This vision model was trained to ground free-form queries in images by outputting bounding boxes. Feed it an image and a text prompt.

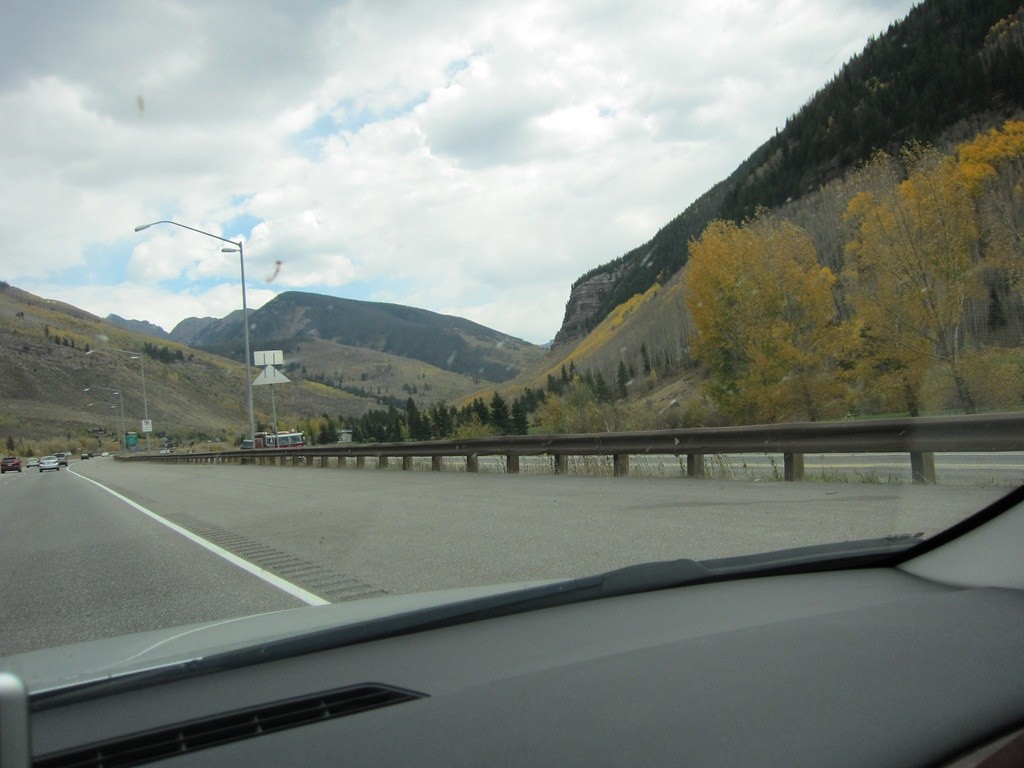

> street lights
[134,220,256,448]
[85,346,150,449]
[89,402,120,451]
[84,385,126,456]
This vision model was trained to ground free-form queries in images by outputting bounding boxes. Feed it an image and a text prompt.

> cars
[102,451,109,457]
[81,454,89,460]
[53,453,67,467]
[39,456,60,473]
[88,451,94,458]
[27,458,39,468]
[1,457,24,473]
